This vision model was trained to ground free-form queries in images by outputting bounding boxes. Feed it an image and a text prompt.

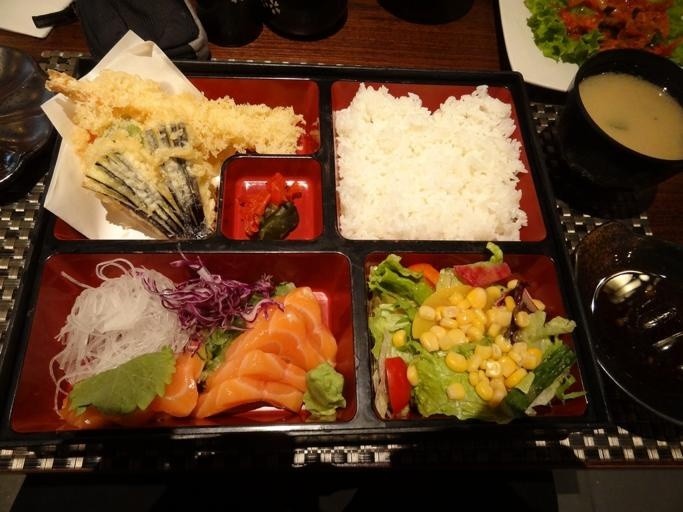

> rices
[331,81,529,240]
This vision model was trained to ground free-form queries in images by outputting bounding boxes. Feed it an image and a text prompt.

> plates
[569,219,683,437]
[0,44,53,188]
[0,56,613,442]
[498,0,683,92]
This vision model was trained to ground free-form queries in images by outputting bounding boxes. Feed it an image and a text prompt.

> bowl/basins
[558,47,683,189]
